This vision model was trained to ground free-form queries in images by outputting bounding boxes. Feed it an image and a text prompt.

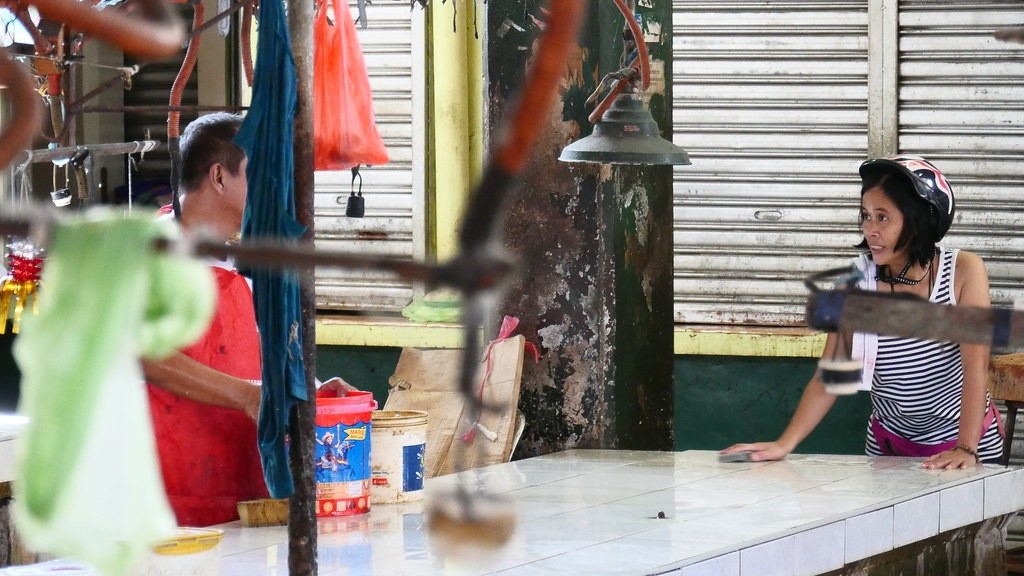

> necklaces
[874,259,932,285]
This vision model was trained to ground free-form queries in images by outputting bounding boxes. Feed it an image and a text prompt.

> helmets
[859,153,955,242]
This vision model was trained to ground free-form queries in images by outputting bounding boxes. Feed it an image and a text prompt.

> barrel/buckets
[371,409,429,505]
[314,390,378,516]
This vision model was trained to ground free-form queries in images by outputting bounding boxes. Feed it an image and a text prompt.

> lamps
[558,62,693,165]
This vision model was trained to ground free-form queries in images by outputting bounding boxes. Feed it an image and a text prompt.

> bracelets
[952,444,979,463]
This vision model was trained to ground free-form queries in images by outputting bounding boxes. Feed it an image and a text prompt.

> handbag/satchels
[873,403,1005,458]
[313,1,390,171]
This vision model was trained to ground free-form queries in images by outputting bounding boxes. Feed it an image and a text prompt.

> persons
[718,155,1006,471]
[137,111,359,527]
